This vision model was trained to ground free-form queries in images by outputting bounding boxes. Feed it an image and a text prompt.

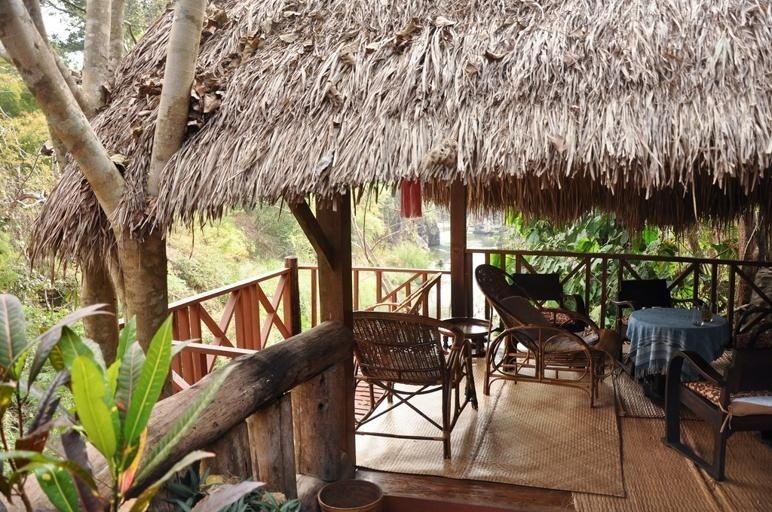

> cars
[692,302,714,326]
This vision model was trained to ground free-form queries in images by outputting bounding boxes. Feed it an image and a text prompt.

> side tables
[438,318,496,357]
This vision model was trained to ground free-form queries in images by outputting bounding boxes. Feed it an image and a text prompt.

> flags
[317,478,384,512]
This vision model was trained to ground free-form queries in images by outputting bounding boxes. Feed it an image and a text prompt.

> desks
[625,309,727,403]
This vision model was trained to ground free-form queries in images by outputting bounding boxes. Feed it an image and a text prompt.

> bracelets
[317,478,384,512]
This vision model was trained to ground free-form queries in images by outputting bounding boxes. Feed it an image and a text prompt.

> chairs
[610,279,707,381]
[660,306,771,480]
[475,265,605,408]
[352,311,478,459]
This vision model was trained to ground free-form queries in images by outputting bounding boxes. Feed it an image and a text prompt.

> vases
[317,478,384,512]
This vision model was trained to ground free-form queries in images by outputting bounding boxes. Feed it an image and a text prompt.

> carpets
[613,366,703,419]
[572,418,770,512]
[357,355,518,479]
[465,358,626,497]
